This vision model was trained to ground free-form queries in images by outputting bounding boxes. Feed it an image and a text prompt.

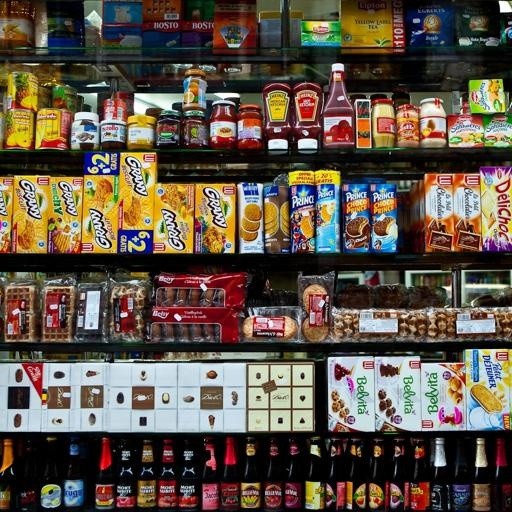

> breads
[74,281,111,346]
[40,284,77,345]
[107,280,148,343]
[150,288,224,343]
[2,280,41,345]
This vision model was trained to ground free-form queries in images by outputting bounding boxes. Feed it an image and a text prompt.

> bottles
[180,109,210,149]
[492,437,512,512]
[177,439,199,510]
[262,436,285,512]
[303,437,327,512]
[368,437,391,512]
[210,99,235,149]
[219,436,242,512]
[155,109,183,149]
[452,437,473,512]
[38,436,63,512]
[136,438,157,510]
[0,438,17,512]
[17,437,39,512]
[409,438,431,512]
[372,97,395,150]
[198,436,221,512]
[472,437,493,512]
[322,61,357,151]
[182,67,208,112]
[63,437,87,511]
[293,82,321,151]
[114,438,137,511]
[99,118,127,150]
[389,438,410,512]
[93,436,117,512]
[127,114,157,150]
[239,436,265,512]
[156,439,179,509]
[325,438,348,512]
[262,83,292,150]
[395,104,421,148]
[282,437,304,511]
[419,96,448,149]
[236,102,264,149]
[430,437,453,512]
[345,438,368,512]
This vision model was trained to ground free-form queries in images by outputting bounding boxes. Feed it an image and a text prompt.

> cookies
[124,196,142,226]
[280,200,290,238]
[332,391,356,429]
[302,284,332,343]
[242,314,298,341]
[468,383,506,417]
[300,216,314,239]
[346,216,370,247]
[202,226,224,254]
[52,232,70,253]
[448,376,462,405]
[240,204,263,241]
[93,178,113,209]
[17,219,35,249]
[374,216,395,236]
[322,204,331,223]
[263,202,280,240]
[378,389,403,430]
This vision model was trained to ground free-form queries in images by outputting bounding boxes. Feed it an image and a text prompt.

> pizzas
[160,184,182,211]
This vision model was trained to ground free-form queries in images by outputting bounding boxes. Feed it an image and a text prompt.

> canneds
[37,84,52,110]
[34,107,72,151]
[51,84,78,114]
[70,111,100,151]
[5,69,39,113]
[2,105,36,150]
[77,95,84,111]
